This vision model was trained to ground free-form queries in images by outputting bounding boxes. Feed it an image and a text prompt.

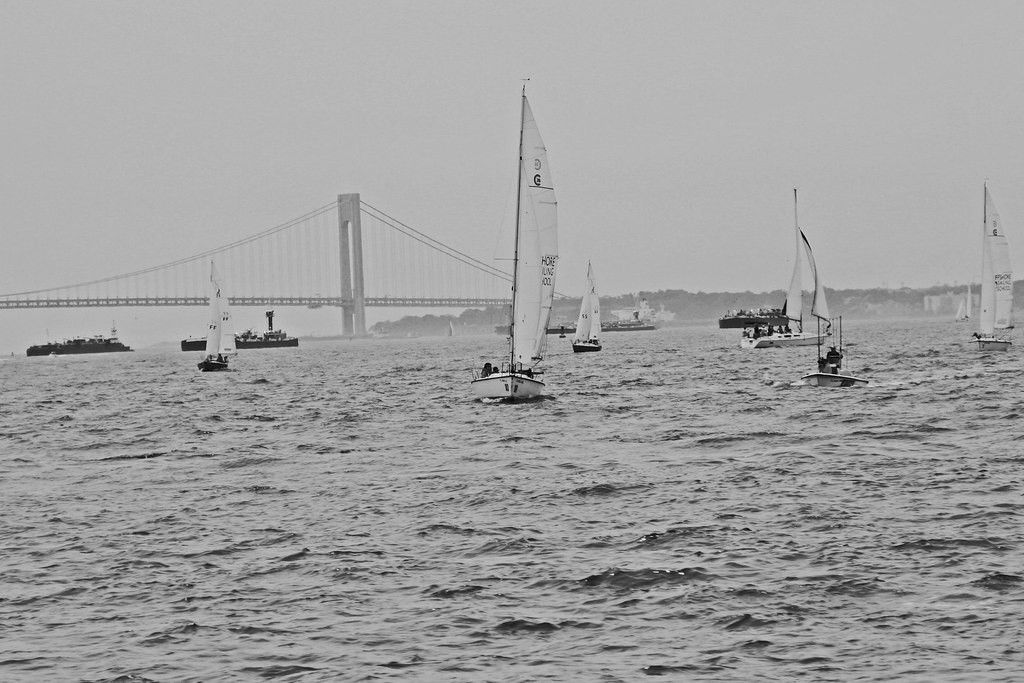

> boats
[180,310,300,354]
[491,318,656,336]
[26,333,135,356]
[718,307,790,329]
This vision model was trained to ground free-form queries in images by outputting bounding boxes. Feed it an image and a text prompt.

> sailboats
[799,226,869,390]
[740,187,834,353]
[572,259,605,352]
[966,180,1014,352]
[469,77,561,405]
[197,257,233,372]
[956,286,973,322]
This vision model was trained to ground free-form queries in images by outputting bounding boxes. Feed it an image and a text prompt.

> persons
[217,354,229,363]
[481,363,499,378]
[743,323,792,339]
[827,346,843,360]
